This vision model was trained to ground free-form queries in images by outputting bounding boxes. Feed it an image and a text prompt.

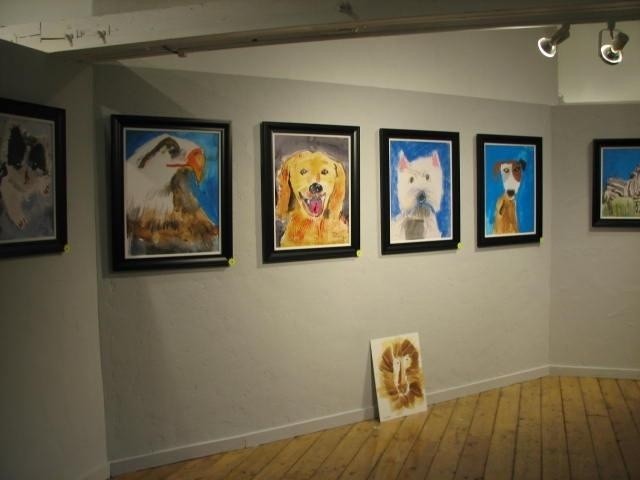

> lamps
[537,22,575,57]
[599,18,630,65]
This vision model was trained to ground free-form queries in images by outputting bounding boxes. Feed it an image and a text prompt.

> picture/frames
[259,121,361,264]
[0,97,67,259]
[593,139,640,227]
[379,128,461,254]
[109,114,233,271]
[476,132,543,248]
